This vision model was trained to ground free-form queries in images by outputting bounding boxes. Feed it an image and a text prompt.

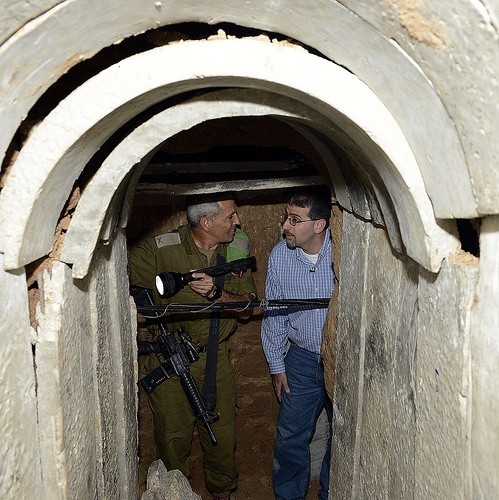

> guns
[129,284,220,447]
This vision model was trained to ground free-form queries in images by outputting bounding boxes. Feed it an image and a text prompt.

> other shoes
[212,490,231,500]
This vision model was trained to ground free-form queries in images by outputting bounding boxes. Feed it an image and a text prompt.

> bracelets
[205,285,216,298]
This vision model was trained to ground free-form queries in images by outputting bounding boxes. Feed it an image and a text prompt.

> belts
[195,346,207,353]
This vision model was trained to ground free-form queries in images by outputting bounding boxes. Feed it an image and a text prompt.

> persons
[128,195,257,500]
[261,187,337,500]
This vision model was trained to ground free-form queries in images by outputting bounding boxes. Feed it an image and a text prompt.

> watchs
[207,284,223,302]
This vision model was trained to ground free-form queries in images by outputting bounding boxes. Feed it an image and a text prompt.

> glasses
[279,214,320,226]
